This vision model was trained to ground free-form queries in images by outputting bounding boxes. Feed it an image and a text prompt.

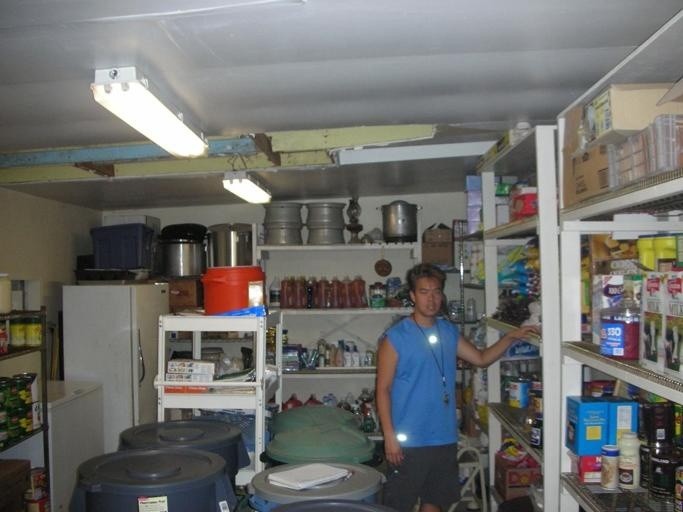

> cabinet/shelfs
[553,11,683,512]
[155,312,284,484]
[255,238,421,443]
[1,305,58,510]
[482,123,557,511]
[446,219,487,480]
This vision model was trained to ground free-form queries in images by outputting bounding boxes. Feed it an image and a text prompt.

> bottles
[466,296,476,320]
[618,430,642,489]
[269,273,367,309]
[0,272,13,316]
[0,311,43,359]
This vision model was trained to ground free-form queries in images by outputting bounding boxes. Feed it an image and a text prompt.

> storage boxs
[421,223,456,267]
[494,440,542,507]
[565,395,638,484]
[561,74,683,208]
[89,222,158,277]
[589,258,682,384]
[166,277,202,312]
[0,459,33,508]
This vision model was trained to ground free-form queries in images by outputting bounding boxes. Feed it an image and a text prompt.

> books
[266,461,354,492]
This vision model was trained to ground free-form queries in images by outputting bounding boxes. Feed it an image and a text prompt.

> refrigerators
[61,282,169,455]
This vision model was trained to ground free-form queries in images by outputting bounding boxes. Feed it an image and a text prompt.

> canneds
[25,316,43,348]
[9,316,25,352]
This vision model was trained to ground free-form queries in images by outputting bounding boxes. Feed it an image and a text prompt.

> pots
[201,224,252,266]
[375,199,422,242]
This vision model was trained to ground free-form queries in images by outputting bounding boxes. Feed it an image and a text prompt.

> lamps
[222,151,275,206]
[91,67,207,160]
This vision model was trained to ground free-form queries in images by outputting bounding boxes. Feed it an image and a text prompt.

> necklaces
[410,313,450,410]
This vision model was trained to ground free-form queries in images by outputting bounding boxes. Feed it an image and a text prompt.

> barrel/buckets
[206,222,252,267]
[117,418,251,468]
[250,463,387,511]
[68,448,239,511]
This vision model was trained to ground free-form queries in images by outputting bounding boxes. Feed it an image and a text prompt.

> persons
[374,261,540,512]
